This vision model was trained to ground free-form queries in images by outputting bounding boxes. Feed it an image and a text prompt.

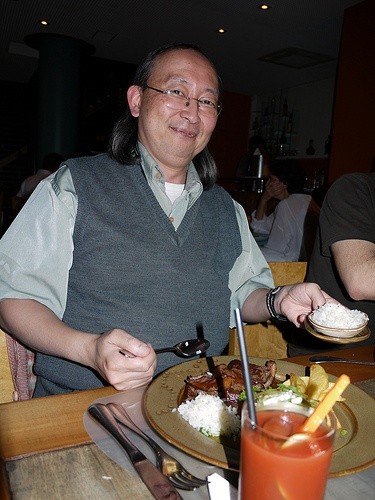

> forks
[107,402,211,491]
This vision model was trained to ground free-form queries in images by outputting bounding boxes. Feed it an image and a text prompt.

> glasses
[141,84,224,118]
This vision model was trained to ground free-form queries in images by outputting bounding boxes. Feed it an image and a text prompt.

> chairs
[0,329,38,405]
[227,261,309,360]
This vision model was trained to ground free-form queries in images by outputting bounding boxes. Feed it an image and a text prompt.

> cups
[238,391,336,500]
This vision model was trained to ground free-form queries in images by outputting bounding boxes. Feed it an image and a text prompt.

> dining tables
[0,345,375,500]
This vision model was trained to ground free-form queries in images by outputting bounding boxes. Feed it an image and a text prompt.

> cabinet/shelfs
[248,94,298,156]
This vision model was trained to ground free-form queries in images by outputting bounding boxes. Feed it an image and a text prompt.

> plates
[305,319,371,343]
[143,355,375,478]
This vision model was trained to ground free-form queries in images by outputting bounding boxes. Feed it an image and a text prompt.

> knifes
[87,403,184,500]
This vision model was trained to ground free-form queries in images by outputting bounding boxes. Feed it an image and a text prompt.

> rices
[312,302,369,329]
[170,391,241,436]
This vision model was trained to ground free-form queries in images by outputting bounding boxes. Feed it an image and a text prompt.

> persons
[288,171,375,356]
[17,153,64,197]
[0,42,352,399]
[251,158,320,262]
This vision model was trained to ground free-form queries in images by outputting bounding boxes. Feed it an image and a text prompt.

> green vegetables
[238,382,321,409]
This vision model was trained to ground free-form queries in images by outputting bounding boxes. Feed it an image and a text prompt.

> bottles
[305,138,316,155]
[324,134,330,155]
[242,162,327,193]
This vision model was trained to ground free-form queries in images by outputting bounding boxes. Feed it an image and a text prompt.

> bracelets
[266,285,287,321]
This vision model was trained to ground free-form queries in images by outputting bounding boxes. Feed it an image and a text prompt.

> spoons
[118,338,210,359]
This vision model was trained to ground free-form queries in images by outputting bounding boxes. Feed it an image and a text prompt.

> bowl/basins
[308,310,369,338]
